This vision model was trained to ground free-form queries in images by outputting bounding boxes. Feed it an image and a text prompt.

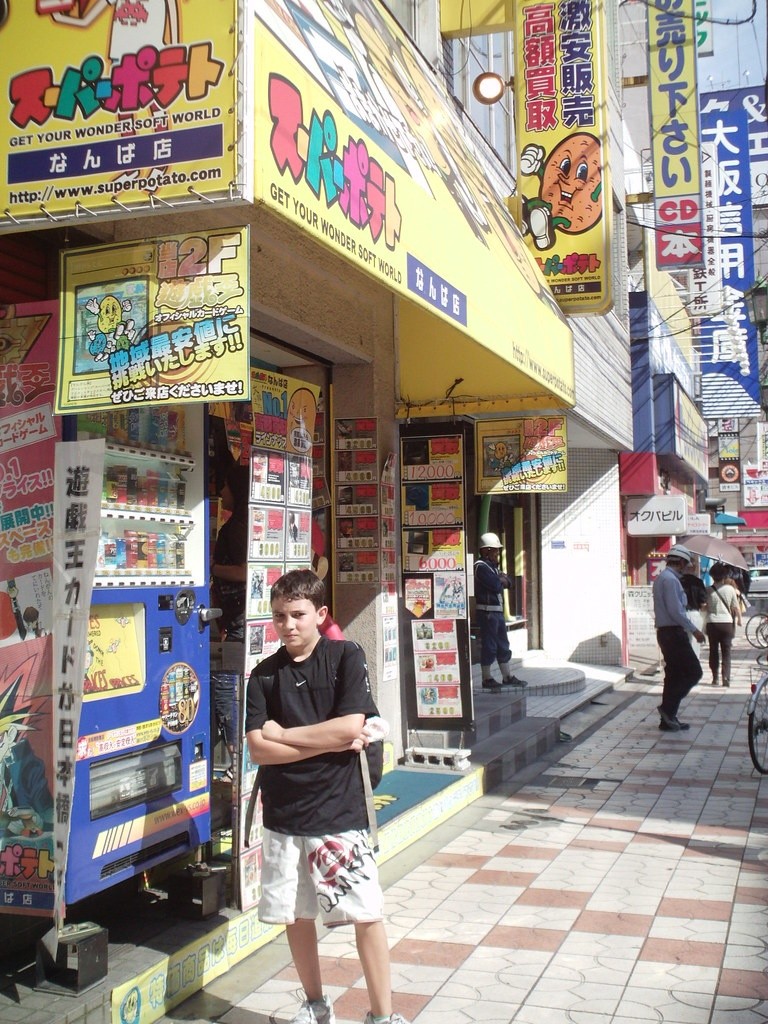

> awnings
[714,513,747,526]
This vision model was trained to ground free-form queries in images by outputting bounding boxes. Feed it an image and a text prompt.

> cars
[747,567,768,593]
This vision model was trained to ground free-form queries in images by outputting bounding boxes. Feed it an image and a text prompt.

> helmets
[478,533,503,549]
[667,544,694,568]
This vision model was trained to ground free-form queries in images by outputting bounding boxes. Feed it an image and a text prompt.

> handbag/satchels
[739,600,746,614]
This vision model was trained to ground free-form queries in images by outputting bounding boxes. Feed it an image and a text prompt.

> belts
[684,608,699,612]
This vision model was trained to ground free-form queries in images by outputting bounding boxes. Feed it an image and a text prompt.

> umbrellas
[676,535,749,571]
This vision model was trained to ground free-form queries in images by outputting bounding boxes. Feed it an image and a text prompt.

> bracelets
[211,563,216,576]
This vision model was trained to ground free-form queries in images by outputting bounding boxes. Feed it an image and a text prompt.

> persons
[245,569,412,1024]
[210,464,250,784]
[653,544,751,731]
[474,533,528,688]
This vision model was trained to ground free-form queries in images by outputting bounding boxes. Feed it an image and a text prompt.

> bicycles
[744,613,768,776]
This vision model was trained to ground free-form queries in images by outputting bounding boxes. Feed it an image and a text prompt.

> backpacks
[253,639,386,791]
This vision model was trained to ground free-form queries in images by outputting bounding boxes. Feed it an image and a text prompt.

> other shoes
[481,679,502,689]
[712,680,719,684]
[658,718,690,730]
[502,676,528,687]
[723,675,729,686]
[657,705,681,732]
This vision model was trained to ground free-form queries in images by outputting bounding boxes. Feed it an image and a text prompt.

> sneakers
[364,1010,411,1024]
[268,989,336,1024]
